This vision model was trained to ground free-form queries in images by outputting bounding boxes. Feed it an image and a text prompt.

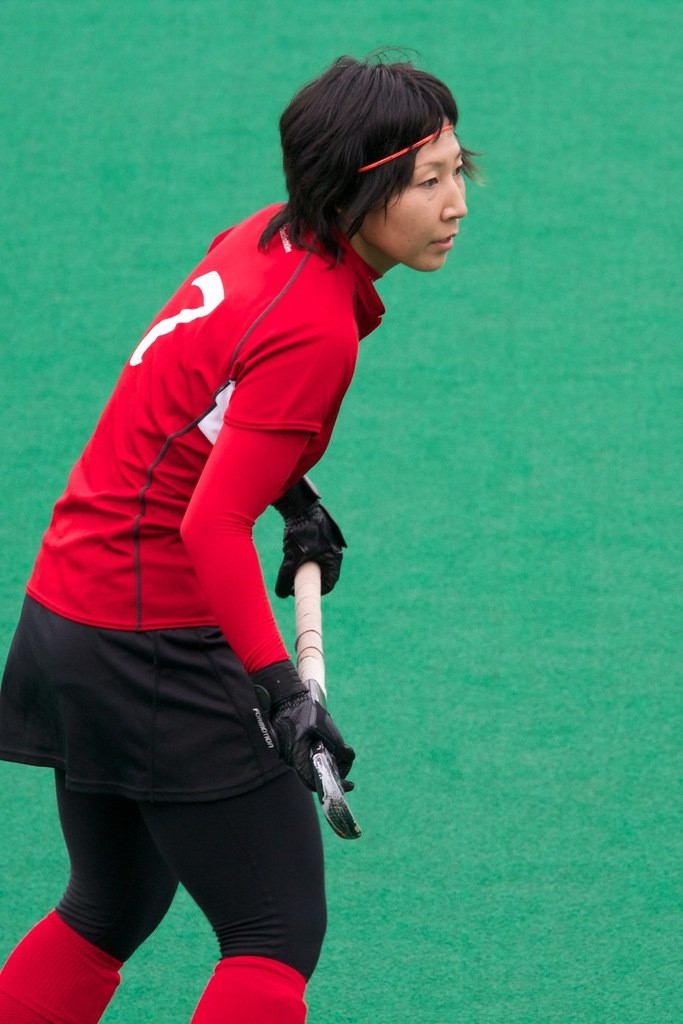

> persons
[0,52,472,1024]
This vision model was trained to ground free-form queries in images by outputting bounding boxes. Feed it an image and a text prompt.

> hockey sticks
[292,560,365,841]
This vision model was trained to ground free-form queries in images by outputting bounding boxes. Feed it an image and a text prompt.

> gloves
[247,659,356,793]
[269,475,348,599]
